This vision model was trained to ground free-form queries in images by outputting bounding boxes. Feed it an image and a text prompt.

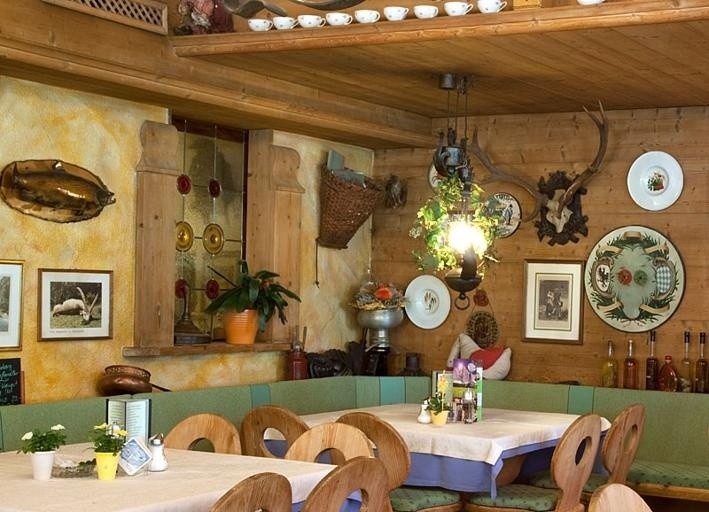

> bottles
[694,332,708,392]
[463,391,474,424]
[657,354,680,392]
[623,339,639,388]
[147,437,168,471]
[601,340,619,387]
[680,330,693,391]
[418,399,431,423]
[645,329,658,389]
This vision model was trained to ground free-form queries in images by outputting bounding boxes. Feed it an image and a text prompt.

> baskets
[318,165,385,250]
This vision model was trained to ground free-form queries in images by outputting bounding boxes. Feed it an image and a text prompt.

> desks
[1,441,339,512]
[295,404,613,511]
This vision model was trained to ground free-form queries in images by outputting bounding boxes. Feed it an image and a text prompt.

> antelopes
[52,284,101,324]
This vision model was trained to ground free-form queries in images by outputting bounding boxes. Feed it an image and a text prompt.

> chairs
[533,403,652,512]
[464,413,602,512]
[284,423,374,480]
[328,412,462,512]
[239,406,318,462]
[161,414,244,457]
[208,472,292,512]
[304,457,390,509]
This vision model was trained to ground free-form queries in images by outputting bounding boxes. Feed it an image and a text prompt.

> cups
[288,351,308,380]
[405,352,420,370]
[245,0,510,34]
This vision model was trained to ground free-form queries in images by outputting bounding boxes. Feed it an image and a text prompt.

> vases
[355,307,404,353]
[31,451,55,481]
[95,450,119,480]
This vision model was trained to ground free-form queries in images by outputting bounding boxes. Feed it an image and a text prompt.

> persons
[505,204,513,224]
[554,288,563,319]
[546,290,554,317]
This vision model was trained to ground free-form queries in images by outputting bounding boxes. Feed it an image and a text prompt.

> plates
[428,148,472,196]
[403,275,450,329]
[627,150,683,210]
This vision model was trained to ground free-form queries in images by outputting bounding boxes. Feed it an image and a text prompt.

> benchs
[591,386,709,512]
[1,375,356,452]
[356,375,592,413]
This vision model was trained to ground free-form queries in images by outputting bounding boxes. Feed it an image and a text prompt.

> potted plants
[204,259,303,345]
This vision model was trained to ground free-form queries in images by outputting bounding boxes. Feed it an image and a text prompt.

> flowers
[15,423,70,454]
[347,275,411,311]
[87,422,129,457]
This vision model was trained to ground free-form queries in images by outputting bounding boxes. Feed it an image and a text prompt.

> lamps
[434,73,484,312]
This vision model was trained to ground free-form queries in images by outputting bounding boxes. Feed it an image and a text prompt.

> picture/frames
[37,268,113,341]
[522,258,586,345]
[0,258,25,352]
[583,225,686,333]
